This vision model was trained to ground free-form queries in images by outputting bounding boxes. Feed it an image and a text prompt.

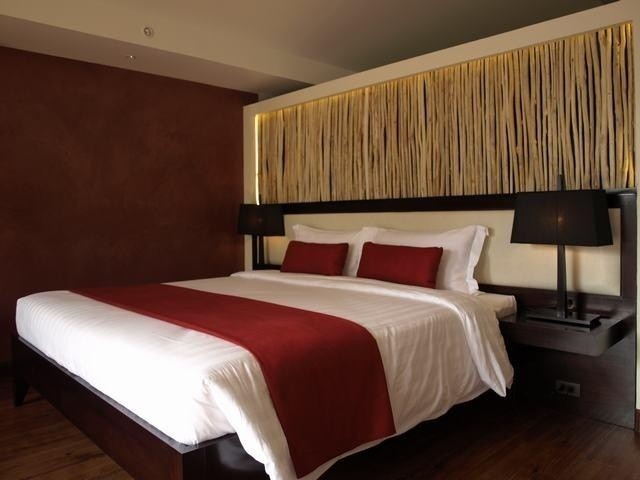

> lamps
[238,201,285,266]
[508,190,615,330]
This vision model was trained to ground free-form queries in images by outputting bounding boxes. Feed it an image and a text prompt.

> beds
[8,271,519,480]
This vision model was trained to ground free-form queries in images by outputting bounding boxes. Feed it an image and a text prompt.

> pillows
[279,222,491,297]
